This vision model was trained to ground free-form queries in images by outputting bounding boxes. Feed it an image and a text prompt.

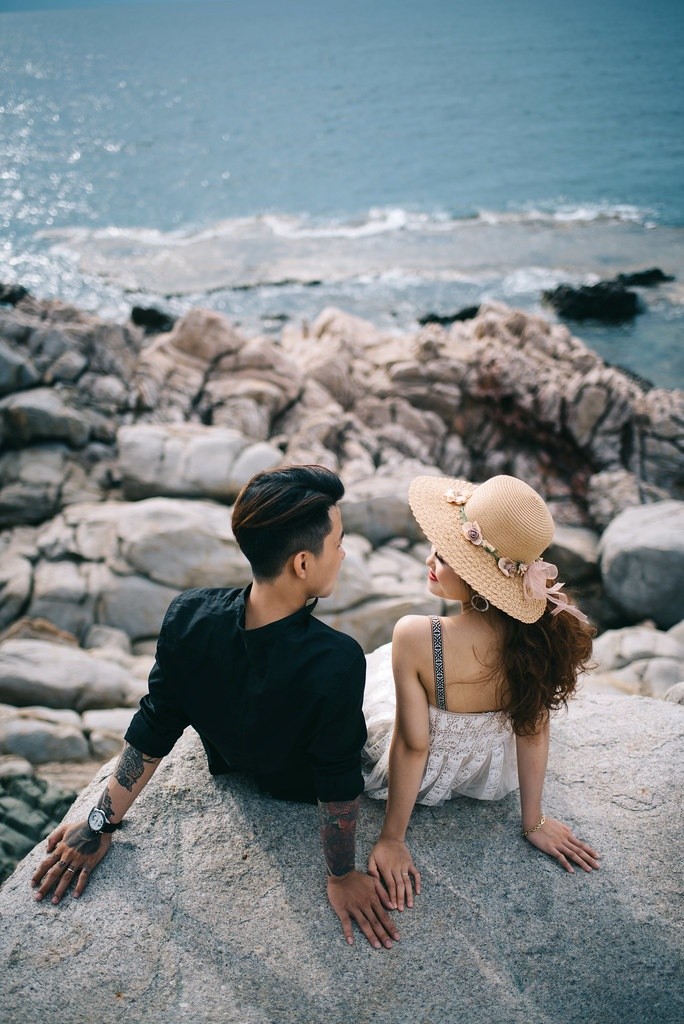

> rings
[401,872,408,876]
[67,867,76,874]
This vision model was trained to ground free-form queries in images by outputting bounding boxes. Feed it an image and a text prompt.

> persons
[28,465,401,950]
[364,473,603,914]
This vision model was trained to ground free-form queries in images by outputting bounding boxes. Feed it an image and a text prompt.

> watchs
[88,806,123,833]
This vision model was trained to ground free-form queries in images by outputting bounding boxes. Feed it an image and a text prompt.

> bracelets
[521,814,545,836]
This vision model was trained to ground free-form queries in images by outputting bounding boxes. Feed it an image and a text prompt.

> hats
[408,474,589,624]
